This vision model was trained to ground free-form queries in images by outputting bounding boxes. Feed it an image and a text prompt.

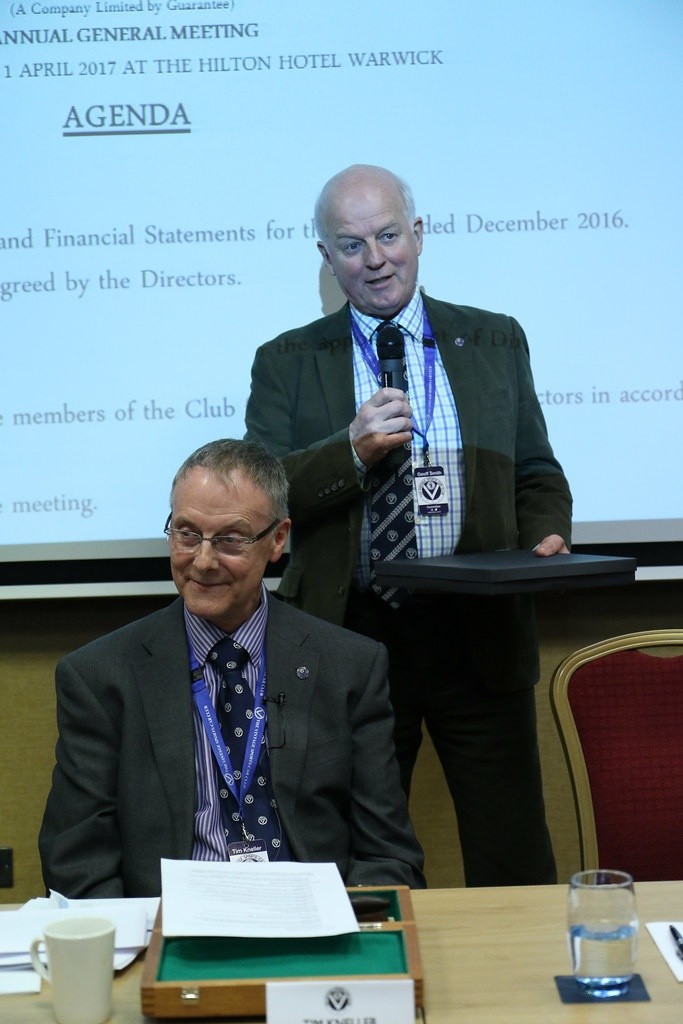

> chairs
[548,626,683,886]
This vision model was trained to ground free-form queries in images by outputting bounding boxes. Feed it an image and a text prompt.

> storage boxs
[139,885,426,1018]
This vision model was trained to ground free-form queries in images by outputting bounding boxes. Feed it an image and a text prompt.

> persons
[243,165,574,890]
[38,437,428,901]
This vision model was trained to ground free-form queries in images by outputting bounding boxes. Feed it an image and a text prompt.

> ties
[204,636,257,848]
[364,320,420,604]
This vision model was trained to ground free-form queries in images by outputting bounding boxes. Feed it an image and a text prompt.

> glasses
[163,511,281,556]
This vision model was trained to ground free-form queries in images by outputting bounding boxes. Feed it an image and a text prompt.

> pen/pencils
[668,923,683,962]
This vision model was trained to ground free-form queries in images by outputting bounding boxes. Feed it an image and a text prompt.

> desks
[0,879,683,1024]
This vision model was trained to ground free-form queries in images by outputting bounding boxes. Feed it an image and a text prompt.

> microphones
[376,326,406,465]
[278,692,287,710]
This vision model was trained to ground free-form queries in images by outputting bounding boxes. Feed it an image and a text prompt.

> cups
[569,870,637,997]
[31,917,115,1024]
